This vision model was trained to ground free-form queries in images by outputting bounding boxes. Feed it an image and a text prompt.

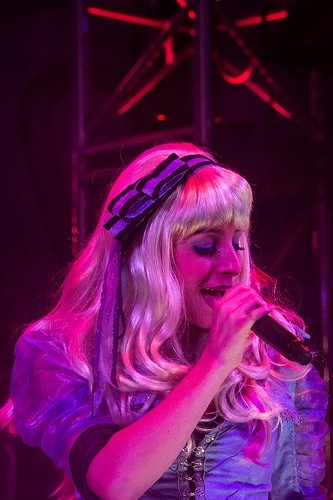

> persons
[1,141,331,500]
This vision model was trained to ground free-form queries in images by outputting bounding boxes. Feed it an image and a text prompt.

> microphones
[250,313,313,365]
